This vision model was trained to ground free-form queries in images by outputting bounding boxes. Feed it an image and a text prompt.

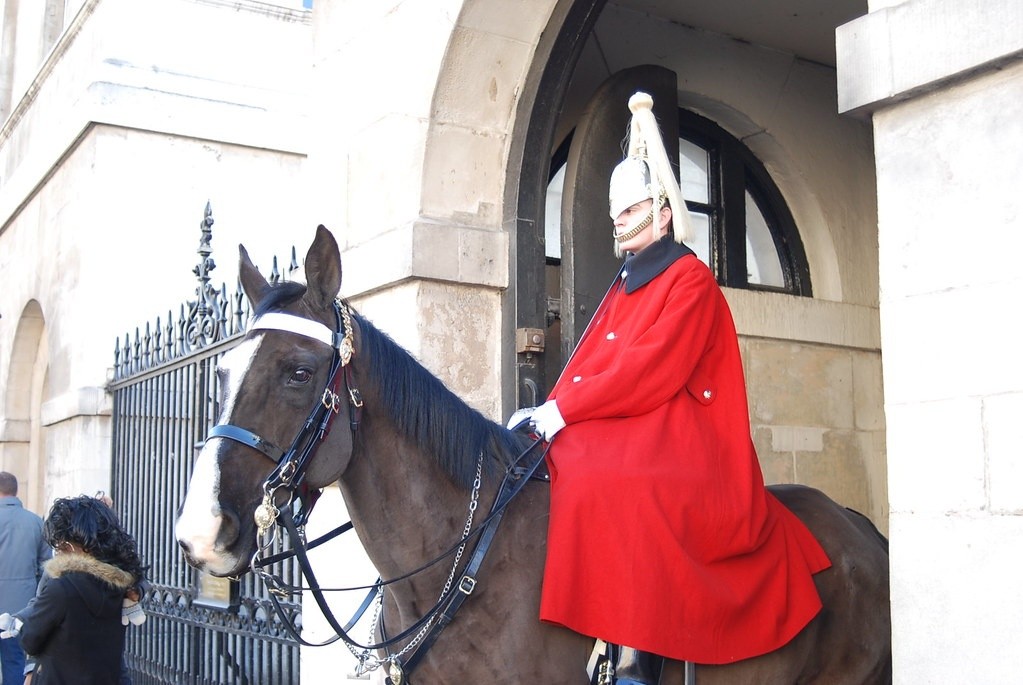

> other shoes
[616,641,665,685]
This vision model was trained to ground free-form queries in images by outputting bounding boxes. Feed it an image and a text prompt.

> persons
[0,469,53,685]
[12,494,151,685]
[526,153,754,685]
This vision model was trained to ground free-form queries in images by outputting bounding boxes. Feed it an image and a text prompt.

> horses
[169,224,891,683]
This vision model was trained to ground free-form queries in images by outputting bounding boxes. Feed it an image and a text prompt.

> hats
[607,90,697,244]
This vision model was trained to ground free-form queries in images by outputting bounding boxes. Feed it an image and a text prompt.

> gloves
[0,613,23,638]
[507,405,537,431]
[531,400,566,442]
[122,599,146,626]
